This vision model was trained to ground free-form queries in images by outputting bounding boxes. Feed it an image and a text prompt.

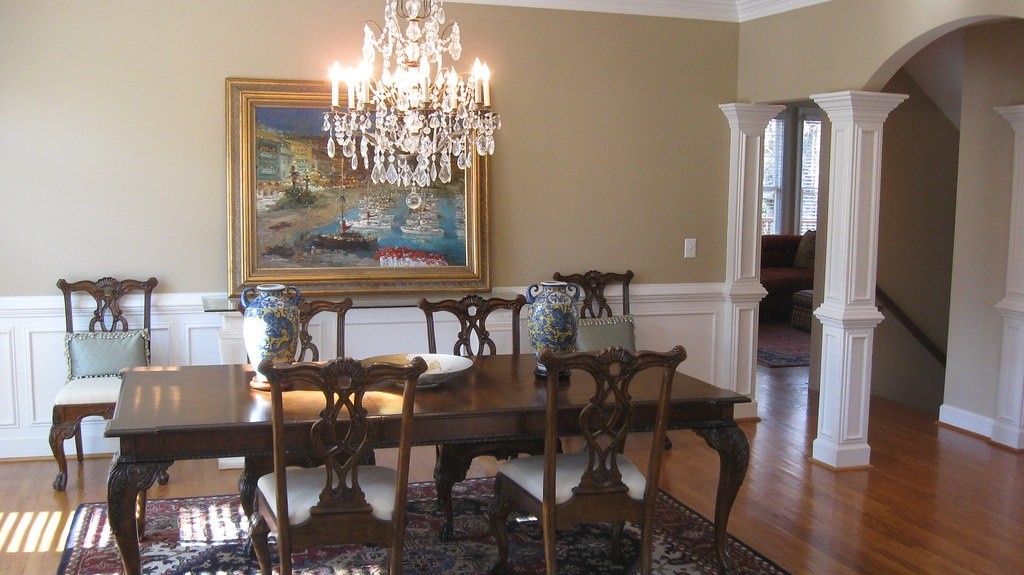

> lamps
[319,0,501,210]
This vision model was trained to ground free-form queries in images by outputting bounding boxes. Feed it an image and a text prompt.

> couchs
[760,230,814,318]
[791,289,812,333]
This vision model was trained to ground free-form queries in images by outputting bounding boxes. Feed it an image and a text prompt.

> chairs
[47,277,168,496]
[480,345,692,573]
[548,269,678,452]
[414,292,562,540]
[227,294,376,535]
[252,355,431,575]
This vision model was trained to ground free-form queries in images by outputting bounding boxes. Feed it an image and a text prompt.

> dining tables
[97,341,757,572]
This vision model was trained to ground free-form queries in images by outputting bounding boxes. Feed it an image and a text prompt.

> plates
[363,352,475,388]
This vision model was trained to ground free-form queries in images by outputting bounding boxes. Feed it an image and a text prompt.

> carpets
[55,480,792,575]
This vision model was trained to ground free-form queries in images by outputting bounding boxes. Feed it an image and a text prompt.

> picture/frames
[225,74,494,300]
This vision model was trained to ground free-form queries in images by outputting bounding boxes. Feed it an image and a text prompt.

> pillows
[793,229,816,270]
